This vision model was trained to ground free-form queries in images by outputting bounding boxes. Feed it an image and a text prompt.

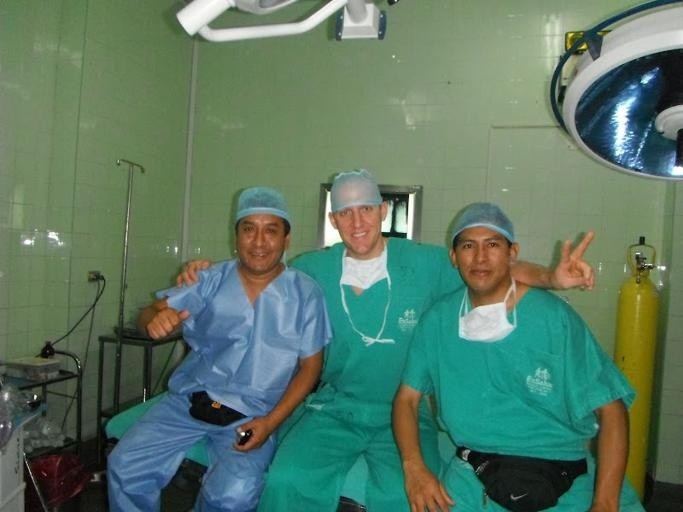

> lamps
[548,1,683,184]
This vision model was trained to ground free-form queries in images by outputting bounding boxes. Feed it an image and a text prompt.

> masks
[457,300,516,342]
[339,251,390,289]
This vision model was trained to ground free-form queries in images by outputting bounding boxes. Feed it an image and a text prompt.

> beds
[104,388,457,512]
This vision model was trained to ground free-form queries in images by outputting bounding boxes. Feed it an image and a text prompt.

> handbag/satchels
[187,389,247,427]
[454,445,589,512]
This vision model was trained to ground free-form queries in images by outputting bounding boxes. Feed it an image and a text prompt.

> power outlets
[88,270,101,284]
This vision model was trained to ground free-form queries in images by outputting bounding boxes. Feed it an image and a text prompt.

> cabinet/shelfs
[0,348,86,463]
[95,331,188,465]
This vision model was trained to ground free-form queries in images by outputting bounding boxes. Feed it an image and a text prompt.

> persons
[384,201,646,512]
[165,164,598,511]
[90,184,336,512]
